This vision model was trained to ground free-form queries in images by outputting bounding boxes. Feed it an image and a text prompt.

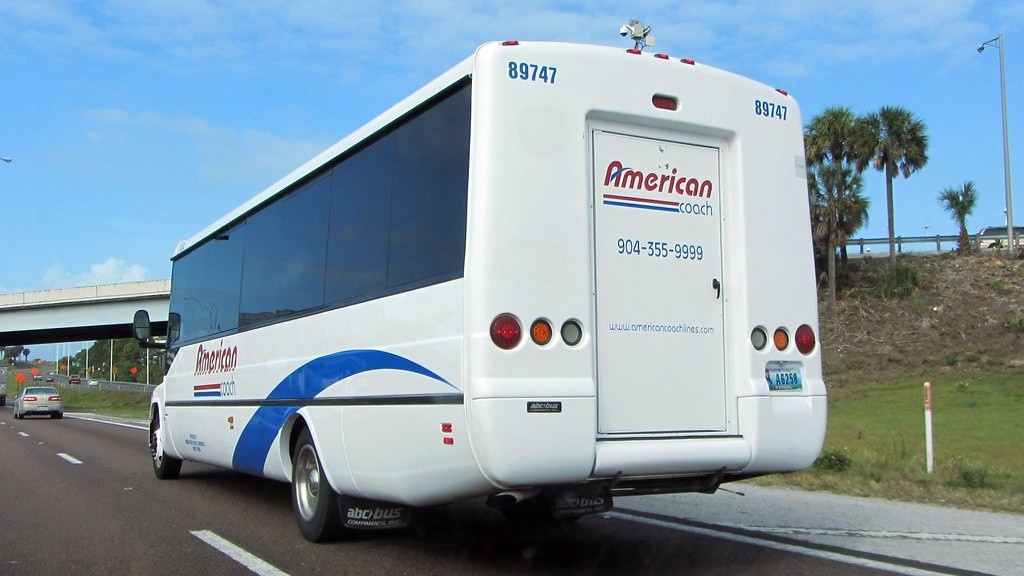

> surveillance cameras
[620,27,628,37]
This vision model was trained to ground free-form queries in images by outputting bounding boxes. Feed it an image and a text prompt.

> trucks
[0,366,9,406]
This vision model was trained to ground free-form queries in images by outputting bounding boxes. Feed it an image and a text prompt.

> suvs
[68,374,81,384]
[968,226,1024,250]
[33,374,43,381]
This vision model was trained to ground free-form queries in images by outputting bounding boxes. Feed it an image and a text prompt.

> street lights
[977,32,1015,257]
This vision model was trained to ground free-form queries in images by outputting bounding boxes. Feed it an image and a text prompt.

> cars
[13,386,64,420]
[45,377,54,382]
[87,379,99,387]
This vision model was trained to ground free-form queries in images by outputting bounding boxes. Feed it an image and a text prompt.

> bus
[130,20,830,546]
